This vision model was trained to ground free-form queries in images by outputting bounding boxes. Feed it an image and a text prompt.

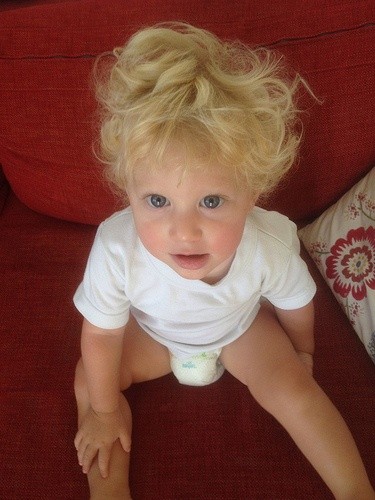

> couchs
[0,0,375,500]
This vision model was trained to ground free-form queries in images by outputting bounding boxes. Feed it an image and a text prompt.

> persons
[73,20,375,500]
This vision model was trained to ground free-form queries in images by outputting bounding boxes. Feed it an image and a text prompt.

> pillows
[296,168,375,363]
